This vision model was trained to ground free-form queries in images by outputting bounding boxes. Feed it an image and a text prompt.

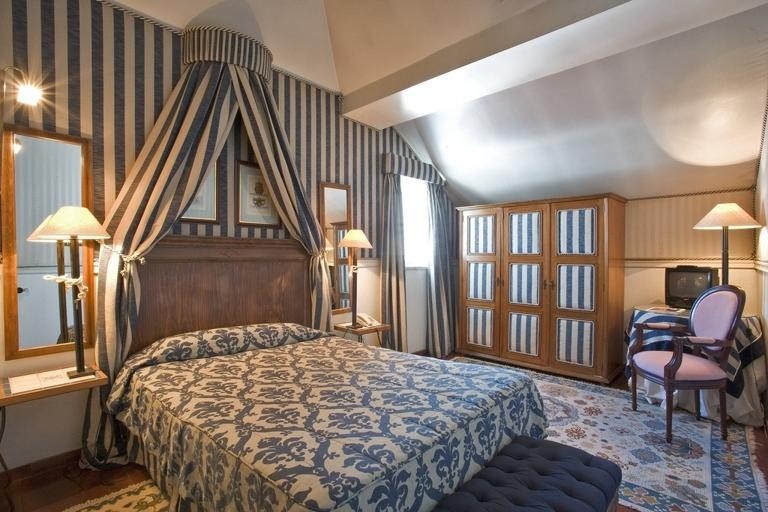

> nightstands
[336,318,391,346]
[2,363,113,511]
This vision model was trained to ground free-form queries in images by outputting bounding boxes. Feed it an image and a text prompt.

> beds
[124,232,546,510]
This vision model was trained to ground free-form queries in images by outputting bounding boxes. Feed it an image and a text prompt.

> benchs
[430,433,621,510]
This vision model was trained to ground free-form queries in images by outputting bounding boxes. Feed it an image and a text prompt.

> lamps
[691,202,762,283]
[1,64,44,116]
[338,229,373,328]
[38,206,108,379]
[24,219,72,344]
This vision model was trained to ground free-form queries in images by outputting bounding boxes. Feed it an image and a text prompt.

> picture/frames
[180,154,221,224]
[236,158,283,230]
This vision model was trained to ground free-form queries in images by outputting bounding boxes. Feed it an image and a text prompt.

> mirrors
[317,182,355,314]
[1,123,97,361]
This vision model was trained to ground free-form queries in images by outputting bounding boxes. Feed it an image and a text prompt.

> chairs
[628,283,746,443]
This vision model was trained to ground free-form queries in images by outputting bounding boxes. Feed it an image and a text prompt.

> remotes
[677,265,697,268]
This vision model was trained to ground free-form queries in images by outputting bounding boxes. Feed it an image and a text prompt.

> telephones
[356,313,381,328]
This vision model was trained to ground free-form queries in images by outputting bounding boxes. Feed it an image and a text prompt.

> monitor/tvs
[665,267,719,310]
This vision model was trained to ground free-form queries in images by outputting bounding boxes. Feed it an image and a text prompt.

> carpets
[63,482,187,512]
[446,353,768,512]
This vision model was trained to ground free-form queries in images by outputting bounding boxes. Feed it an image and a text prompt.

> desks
[625,301,767,427]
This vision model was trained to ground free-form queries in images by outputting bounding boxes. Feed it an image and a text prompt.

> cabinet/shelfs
[331,222,350,308]
[452,192,627,386]
